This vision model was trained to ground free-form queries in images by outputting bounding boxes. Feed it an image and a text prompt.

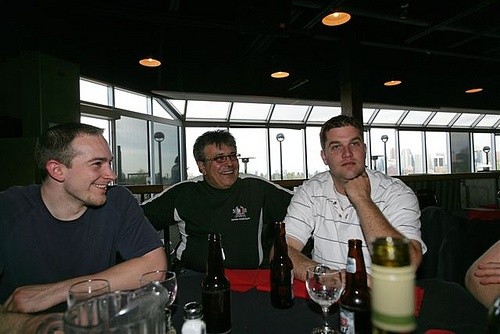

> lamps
[383,72,402,86]
[464,84,483,94]
[320,8,352,27]
[270,65,290,79]
[138,48,161,68]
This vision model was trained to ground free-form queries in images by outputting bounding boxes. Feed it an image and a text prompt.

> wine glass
[304,263,343,334]
[138,270,178,334]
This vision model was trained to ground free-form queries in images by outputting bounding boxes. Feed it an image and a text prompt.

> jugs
[62,289,167,334]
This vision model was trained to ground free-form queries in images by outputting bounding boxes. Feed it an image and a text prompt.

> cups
[66,279,110,310]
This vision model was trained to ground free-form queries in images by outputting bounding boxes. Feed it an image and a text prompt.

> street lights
[483,146,490,165]
[381,135,388,175]
[239,156,254,174]
[371,156,384,171]
[155,132,164,184]
[276,133,284,180]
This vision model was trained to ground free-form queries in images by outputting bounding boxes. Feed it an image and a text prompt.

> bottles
[180,301,206,334]
[368,237,420,334]
[338,239,373,334]
[201,234,232,334]
[271,223,295,309]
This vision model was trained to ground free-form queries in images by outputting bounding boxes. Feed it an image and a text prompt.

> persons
[0,123,169,334]
[269,116,427,293]
[140,129,314,273]
[465,239,500,310]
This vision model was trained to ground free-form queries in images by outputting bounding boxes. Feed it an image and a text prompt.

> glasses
[205,154,241,163]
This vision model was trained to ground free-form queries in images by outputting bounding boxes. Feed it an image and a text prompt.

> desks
[173,272,341,334]
[448,204,500,224]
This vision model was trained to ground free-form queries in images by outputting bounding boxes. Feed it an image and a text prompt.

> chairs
[414,189,439,207]
